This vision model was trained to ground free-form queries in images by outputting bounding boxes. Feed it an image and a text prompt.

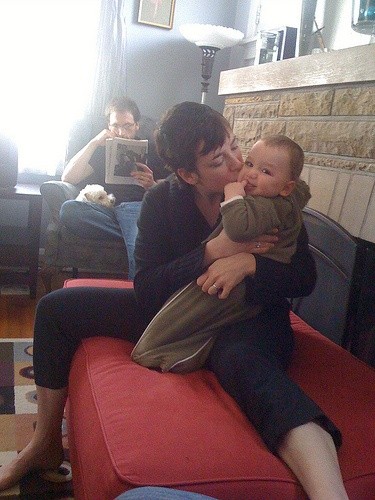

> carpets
[0,337,74,500]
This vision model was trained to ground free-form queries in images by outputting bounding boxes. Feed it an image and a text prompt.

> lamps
[179,24,244,104]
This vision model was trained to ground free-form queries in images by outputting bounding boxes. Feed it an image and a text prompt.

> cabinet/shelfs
[240,26,297,66]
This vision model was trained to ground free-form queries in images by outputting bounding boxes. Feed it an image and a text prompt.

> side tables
[0,183,42,299]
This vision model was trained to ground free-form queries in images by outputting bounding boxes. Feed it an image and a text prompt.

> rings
[212,284,220,289]
[255,241,262,248]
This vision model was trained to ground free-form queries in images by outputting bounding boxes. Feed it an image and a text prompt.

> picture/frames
[137,0,176,30]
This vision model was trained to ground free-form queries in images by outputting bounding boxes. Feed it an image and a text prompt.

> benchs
[61,276,374,499]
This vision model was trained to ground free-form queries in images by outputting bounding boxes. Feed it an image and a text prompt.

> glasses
[110,122,135,131]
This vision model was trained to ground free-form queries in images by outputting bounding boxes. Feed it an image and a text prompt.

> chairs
[40,116,171,294]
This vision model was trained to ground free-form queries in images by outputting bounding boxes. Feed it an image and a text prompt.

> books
[104,138,148,185]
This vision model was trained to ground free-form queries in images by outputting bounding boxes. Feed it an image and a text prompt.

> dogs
[73,182,117,210]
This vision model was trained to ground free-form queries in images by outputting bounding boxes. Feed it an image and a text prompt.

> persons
[59,98,167,281]
[131,135,312,373]
[0,101,349,500]
